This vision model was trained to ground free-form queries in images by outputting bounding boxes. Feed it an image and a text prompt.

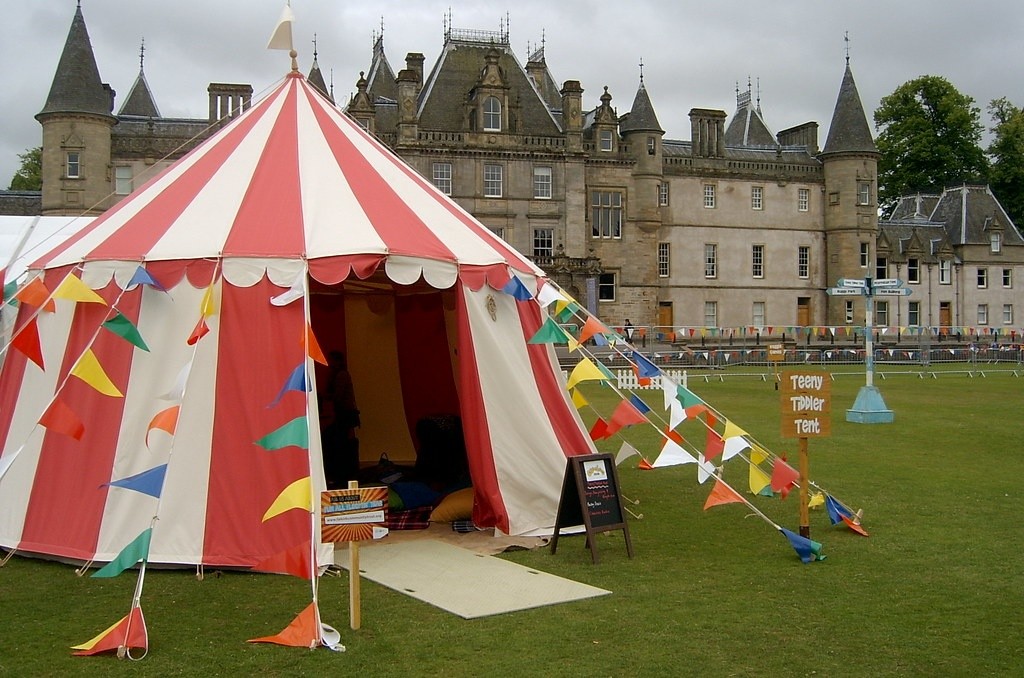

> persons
[624,319,634,343]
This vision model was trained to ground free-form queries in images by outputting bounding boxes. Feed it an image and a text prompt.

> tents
[0,48,601,583]
[1,215,98,272]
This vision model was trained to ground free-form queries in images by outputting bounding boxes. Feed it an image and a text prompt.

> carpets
[335,539,612,620]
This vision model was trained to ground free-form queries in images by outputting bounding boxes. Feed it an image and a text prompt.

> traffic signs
[826,277,913,296]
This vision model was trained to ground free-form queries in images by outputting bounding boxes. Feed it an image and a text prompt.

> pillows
[391,482,444,510]
[427,487,474,523]
[358,482,403,509]
